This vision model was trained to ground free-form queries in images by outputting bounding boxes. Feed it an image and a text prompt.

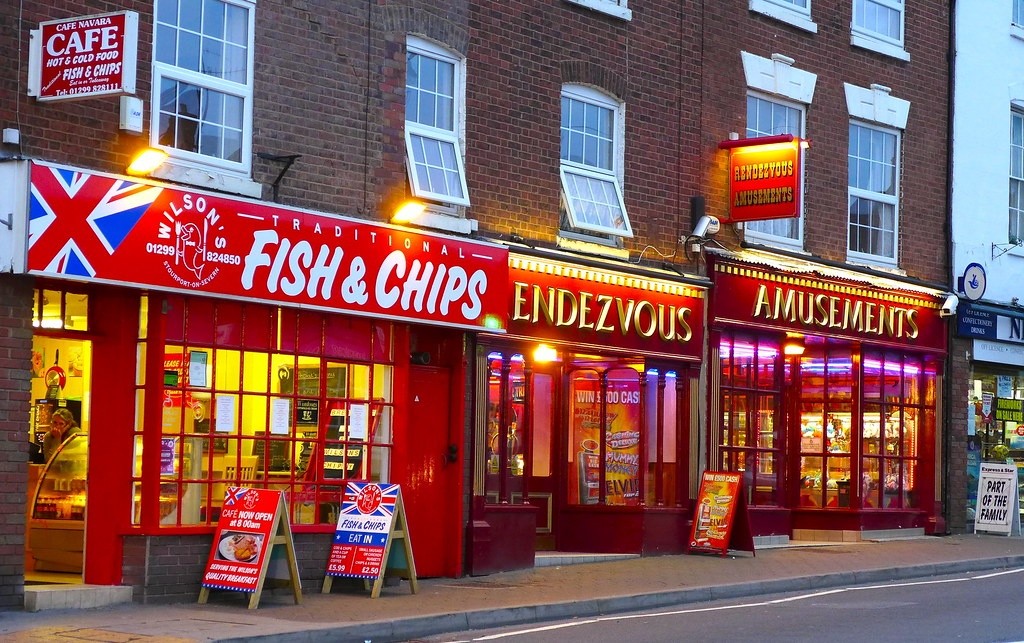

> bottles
[56,497,71,520]
[71,499,86,520]
[43,498,49,519]
[37,497,42,519]
[49,498,56,520]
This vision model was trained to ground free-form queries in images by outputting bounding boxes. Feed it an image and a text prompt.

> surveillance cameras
[692,216,711,238]
[942,296,959,316]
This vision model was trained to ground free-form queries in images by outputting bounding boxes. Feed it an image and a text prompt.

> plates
[219,534,260,561]
[583,439,599,451]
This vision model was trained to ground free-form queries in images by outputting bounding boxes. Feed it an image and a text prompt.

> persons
[43,408,82,474]
